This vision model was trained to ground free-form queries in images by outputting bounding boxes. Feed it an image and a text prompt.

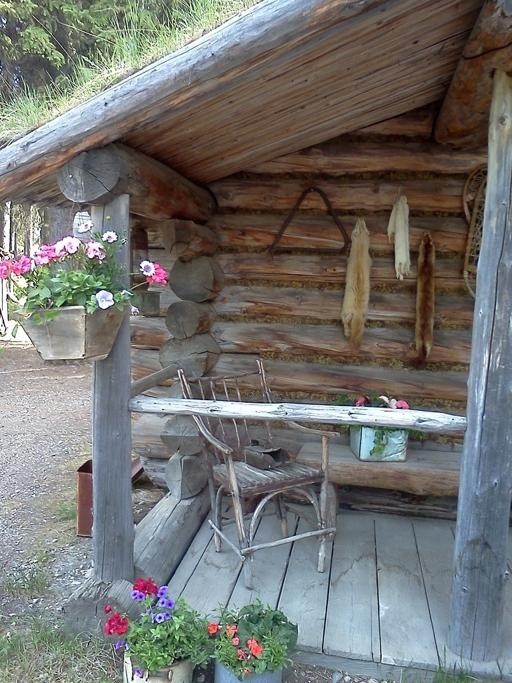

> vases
[11,304,125,364]
[349,428,411,462]
[75,458,145,538]
[123,643,283,683]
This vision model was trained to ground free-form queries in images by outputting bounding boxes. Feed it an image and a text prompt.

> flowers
[102,576,302,681]
[348,394,424,456]
[0,213,169,336]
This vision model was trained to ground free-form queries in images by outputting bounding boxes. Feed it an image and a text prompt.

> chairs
[176,357,342,589]
